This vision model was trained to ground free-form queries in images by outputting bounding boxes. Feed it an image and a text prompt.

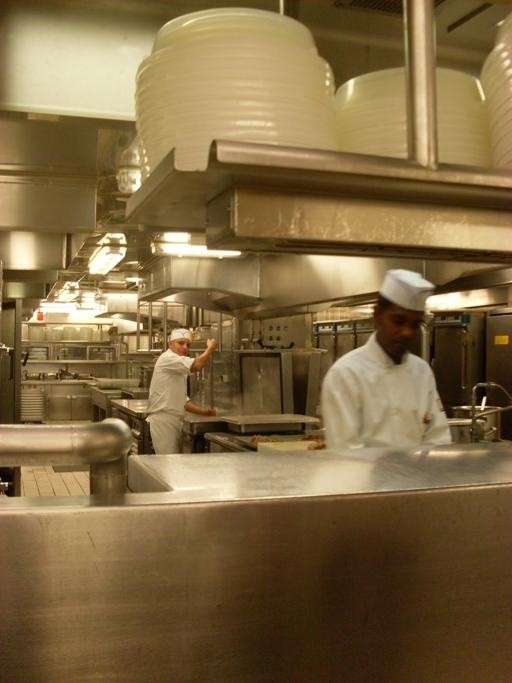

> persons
[319,269,453,449]
[136,327,219,456]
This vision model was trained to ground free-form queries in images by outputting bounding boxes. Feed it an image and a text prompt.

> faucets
[469,381,512,443]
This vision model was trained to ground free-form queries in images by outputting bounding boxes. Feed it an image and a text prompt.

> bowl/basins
[132,8,512,182]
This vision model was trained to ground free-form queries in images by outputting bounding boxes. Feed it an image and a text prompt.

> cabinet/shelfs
[49,393,94,422]
[18,318,120,362]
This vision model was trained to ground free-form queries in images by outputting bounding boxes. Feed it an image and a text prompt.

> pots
[449,406,501,442]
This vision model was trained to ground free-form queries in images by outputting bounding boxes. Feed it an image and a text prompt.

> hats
[378,267,438,314]
[169,327,193,342]
